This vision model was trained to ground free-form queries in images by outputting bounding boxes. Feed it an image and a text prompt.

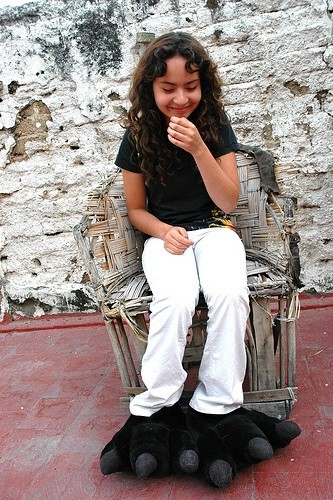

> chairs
[74,143,306,419]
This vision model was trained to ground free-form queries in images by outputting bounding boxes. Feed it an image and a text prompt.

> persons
[97,32,301,487]
